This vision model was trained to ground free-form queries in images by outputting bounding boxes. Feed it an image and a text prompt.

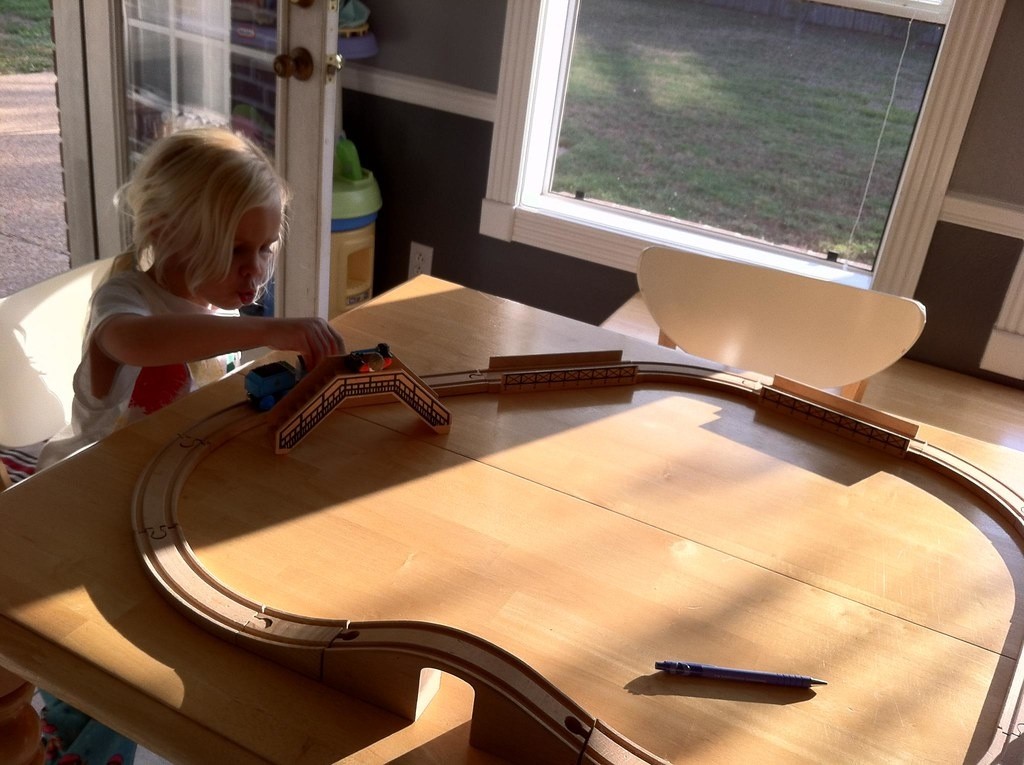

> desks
[1,272,1024,765]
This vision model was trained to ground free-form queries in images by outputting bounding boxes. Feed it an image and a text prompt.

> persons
[35,128,345,765]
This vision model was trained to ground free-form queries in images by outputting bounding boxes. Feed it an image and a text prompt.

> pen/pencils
[653,661,829,689]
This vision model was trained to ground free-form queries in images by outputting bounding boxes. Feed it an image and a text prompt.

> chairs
[635,244,925,399]
